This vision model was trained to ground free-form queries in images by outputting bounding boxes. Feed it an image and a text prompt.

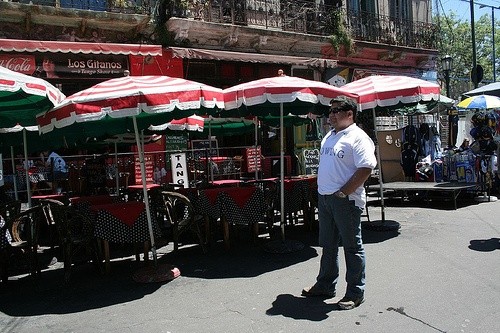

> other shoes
[337,295,366,309]
[302,285,337,298]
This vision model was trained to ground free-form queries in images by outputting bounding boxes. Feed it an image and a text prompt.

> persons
[42,54,59,78]
[302,95,377,310]
[40,148,69,194]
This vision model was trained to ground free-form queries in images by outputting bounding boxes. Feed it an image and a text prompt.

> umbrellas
[36,70,226,260]
[203,69,362,235]
[457,95,500,111]
[340,75,500,139]
[0,65,66,209]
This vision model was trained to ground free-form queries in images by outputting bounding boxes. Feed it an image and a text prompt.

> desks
[264,174,317,230]
[184,185,264,242]
[69,195,163,274]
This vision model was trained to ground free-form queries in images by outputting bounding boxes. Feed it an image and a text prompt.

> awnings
[169,47,337,69]
[0,38,162,56]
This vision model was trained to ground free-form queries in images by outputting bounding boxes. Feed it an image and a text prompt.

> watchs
[339,191,346,198]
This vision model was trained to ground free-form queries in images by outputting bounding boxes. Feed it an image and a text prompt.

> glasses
[328,107,348,114]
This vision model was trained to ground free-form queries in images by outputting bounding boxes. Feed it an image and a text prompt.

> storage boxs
[374,129,408,181]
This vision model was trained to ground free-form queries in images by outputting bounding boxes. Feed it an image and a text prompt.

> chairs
[0,179,371,279]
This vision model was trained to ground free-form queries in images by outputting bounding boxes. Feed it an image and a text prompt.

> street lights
[440,54,454,150]
